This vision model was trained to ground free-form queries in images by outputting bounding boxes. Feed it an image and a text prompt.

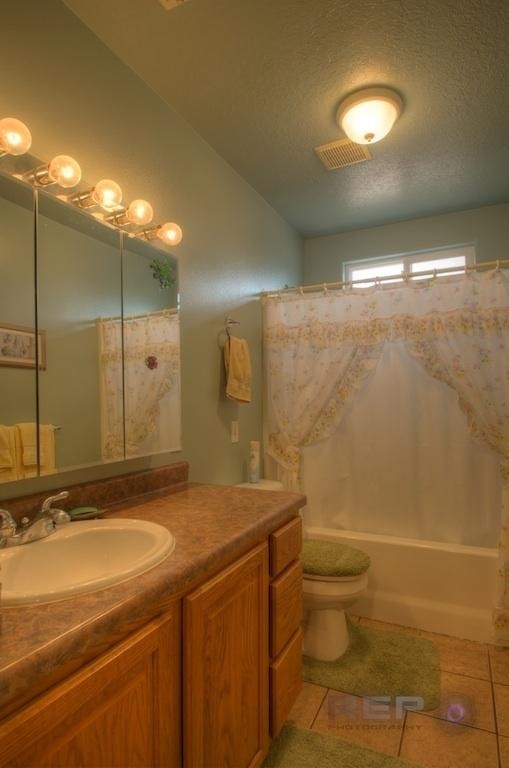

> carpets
[301,616,443,714]
[267,719,428,768]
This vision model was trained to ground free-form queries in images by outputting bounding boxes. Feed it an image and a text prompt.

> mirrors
[0,171,183,502]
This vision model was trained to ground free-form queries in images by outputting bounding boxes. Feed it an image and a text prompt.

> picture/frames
[2,319,48,374]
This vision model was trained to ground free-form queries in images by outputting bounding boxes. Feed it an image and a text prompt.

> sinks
[0,519,175,608]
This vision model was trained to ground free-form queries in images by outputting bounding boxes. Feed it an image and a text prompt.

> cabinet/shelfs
[0,604,179,767]
[180,537,275,768]
[267,511,308,735]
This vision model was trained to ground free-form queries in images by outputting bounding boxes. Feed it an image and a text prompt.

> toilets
[233,480,370,662]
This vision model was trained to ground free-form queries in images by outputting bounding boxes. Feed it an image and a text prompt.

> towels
[225,335,256,409]
[16,420,56,483]
[1,423,21,485]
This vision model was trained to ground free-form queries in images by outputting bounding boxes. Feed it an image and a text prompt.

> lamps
[327,82,412,148]
[0,113,186,248]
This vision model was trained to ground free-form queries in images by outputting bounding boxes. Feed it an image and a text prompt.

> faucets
[22,508,69,545]
